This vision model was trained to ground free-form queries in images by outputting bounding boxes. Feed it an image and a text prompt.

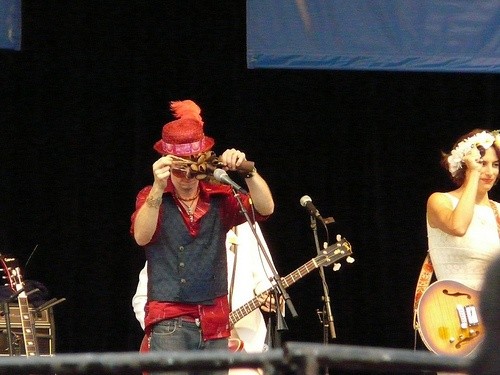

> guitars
[0,254,42,359]
[417,279,486,359]
[140,235,359,375]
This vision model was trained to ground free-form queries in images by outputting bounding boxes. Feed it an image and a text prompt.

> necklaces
[175,187,200,222]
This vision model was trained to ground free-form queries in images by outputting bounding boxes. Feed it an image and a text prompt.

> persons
[130,100,274,375]
[426,129,500,375]
[132,221,285,375]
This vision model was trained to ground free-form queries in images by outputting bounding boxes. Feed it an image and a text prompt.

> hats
[154,100,215,156]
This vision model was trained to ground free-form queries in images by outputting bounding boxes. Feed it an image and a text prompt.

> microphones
[213,168,247,194]
[299,195,323,220]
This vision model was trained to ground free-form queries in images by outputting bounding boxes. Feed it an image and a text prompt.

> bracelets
[146,195,162,208]
[241,166,257,178]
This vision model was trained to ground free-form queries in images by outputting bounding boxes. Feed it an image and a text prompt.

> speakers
[0,329,55,359]
